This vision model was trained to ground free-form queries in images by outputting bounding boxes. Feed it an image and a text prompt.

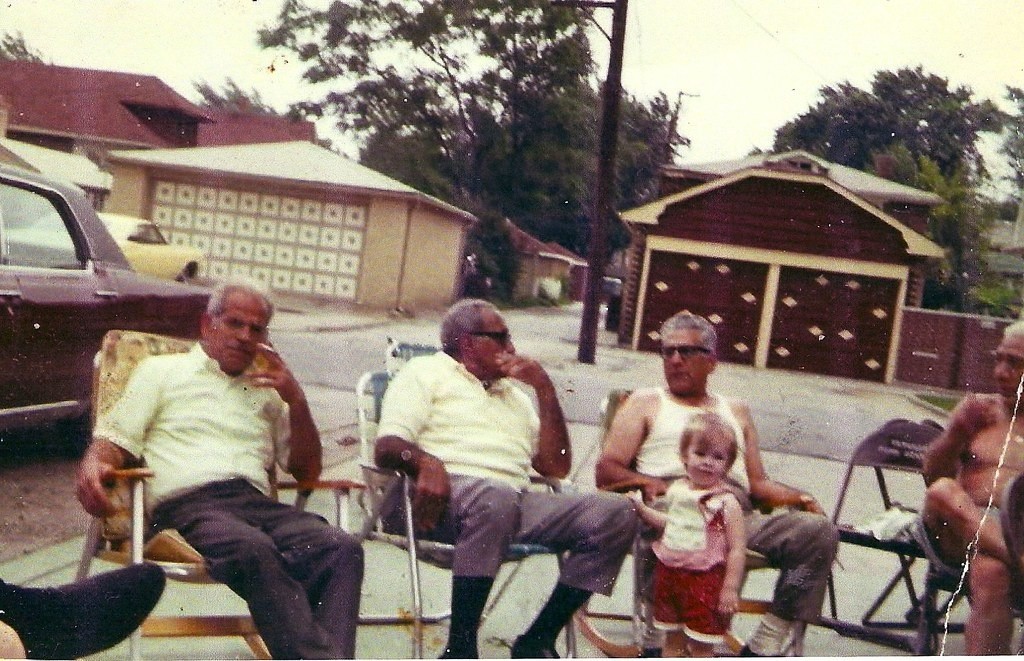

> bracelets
[800,493,813,509]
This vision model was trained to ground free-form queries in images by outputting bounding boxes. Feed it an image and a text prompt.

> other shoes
[639,647,662,658]
[740,645,761,658]
[511,635,560,658]
[1,562,165,660]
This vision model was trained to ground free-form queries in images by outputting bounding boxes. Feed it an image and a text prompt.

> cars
[0,160,218,453]
[2,208,205,286]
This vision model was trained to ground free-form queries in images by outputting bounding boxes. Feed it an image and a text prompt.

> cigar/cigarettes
[258,343,276,353]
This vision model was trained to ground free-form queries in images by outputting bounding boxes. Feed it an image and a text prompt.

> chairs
[73,328,1024,661]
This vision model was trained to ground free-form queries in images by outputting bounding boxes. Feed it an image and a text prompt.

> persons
[375,300,638,658]
[0,561,166,660]
[627,413,746,658]
[596,310,840,658]
[75,278,365,660]
[913,322,1024,655]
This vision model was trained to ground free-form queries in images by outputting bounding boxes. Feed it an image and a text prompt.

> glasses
[659,345,711,360]
[473,331,511,348]
